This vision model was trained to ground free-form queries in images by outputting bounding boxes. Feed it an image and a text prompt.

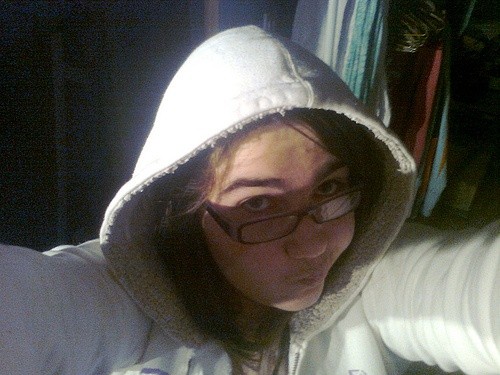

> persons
[1,22,500,375]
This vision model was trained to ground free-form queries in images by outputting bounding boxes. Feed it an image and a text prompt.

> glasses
[202,176,366,245]
